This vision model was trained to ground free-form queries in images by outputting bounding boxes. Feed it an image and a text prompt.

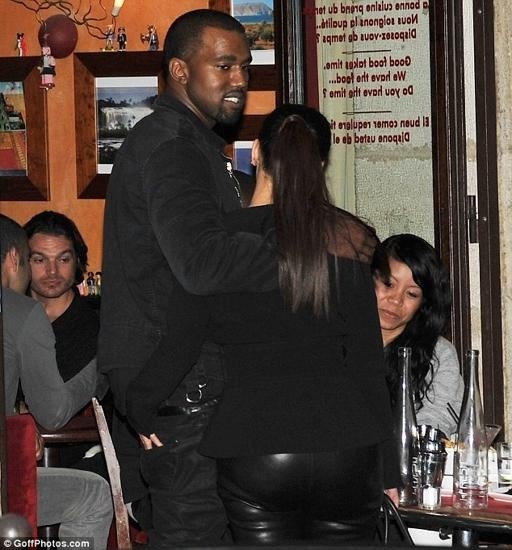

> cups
[496,441,512,491]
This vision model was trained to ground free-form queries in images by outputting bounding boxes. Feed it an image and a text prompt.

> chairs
[1,396,152,549]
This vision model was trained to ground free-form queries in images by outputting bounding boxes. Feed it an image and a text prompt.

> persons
[92,6,381,549]
[10,22,162,92]
[374,233,466,440]
[204,104,405,549]
[15,209,111,483]
[1,214,115,550]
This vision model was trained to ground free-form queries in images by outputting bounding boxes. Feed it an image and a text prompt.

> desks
[399,486,512,547]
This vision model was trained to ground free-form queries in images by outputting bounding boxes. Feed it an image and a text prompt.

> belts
[154,394,221,418]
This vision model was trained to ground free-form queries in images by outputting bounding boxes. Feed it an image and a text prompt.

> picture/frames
[208,0,281,92]
[221,115,278,181]
[0,54,53,203]
[72,50,167,200]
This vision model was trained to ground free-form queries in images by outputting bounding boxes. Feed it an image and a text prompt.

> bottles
[451,348,491,512]
[393,345,420,508]
